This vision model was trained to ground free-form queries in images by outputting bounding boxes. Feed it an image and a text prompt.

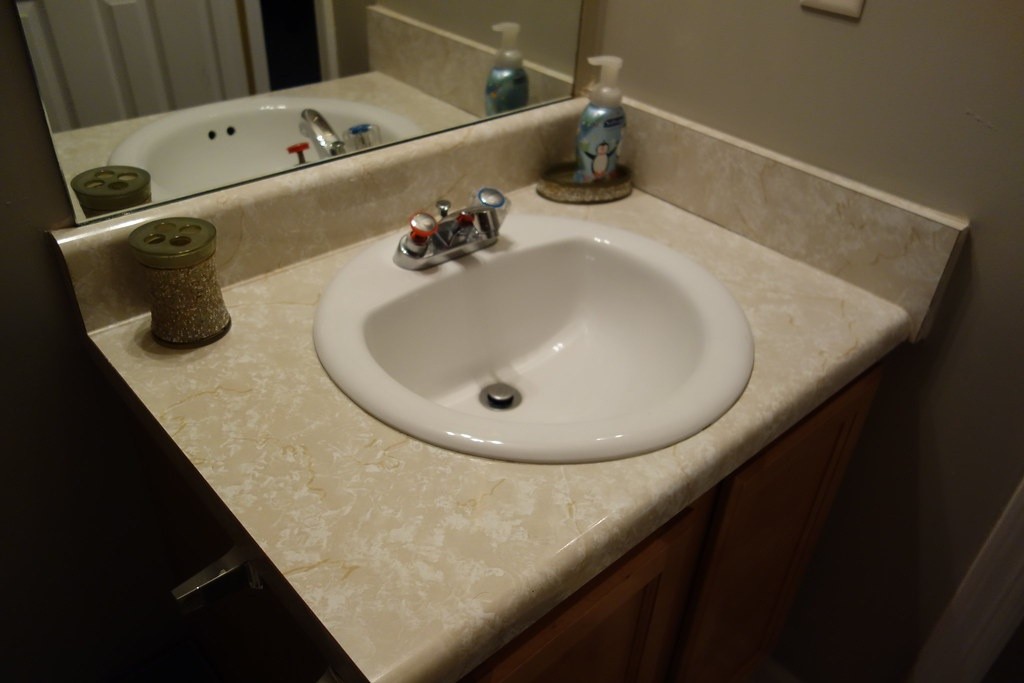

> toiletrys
[573,55,627,185]
[484,21,530,117]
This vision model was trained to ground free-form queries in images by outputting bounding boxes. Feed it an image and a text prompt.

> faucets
[297,107,348,161]
[437,206,500,250]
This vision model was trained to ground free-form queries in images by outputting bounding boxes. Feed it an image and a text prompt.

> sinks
[105,93,426,204]
[312,214,755,465]
